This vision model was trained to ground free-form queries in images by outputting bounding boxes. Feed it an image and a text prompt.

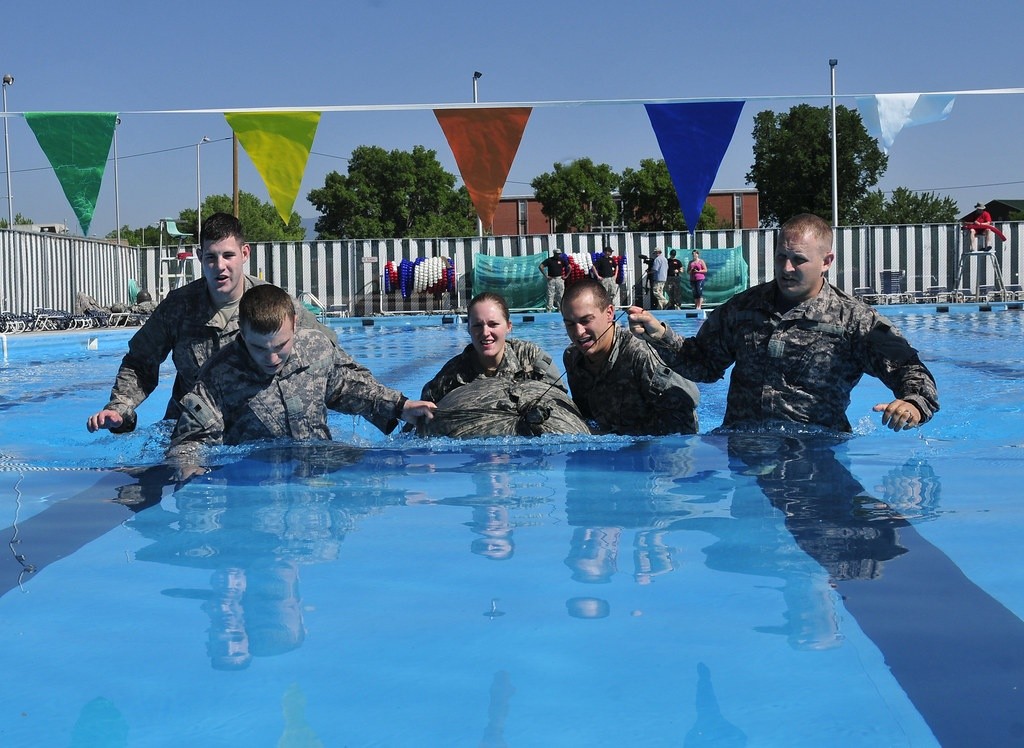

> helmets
[135,291,151,305]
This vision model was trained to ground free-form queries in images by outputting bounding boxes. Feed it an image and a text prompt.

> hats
[604,247,614,252]
[654,246,663,253]
[553,249,562,254]
[974,201,986,209]
[670,250,676,255]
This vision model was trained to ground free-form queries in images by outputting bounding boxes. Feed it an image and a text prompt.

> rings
[905,410,911,416]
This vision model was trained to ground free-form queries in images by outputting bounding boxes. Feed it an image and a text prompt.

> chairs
[854,284,1024,305]
[320,304,349,319]
[0,307,152,335]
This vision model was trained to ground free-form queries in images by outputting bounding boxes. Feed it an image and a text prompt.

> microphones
[638,255,648,259]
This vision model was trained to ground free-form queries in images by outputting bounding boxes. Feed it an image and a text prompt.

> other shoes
[984,246,992,251]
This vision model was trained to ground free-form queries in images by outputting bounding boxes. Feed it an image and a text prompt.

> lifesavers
[384,255,455,299]
[561,252,628,285]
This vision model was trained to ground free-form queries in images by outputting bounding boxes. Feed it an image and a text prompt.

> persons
[592,247,618,300]
[648,247,671,310]
[559,278,700,434]
[419,292,567,401]
[688,250,708,309]
[171,284,438,449]
[627,213,940,432]
[667,249,682,310]
[970,202,992,251]
[87,213,338,434]
[540,248,572,309]
